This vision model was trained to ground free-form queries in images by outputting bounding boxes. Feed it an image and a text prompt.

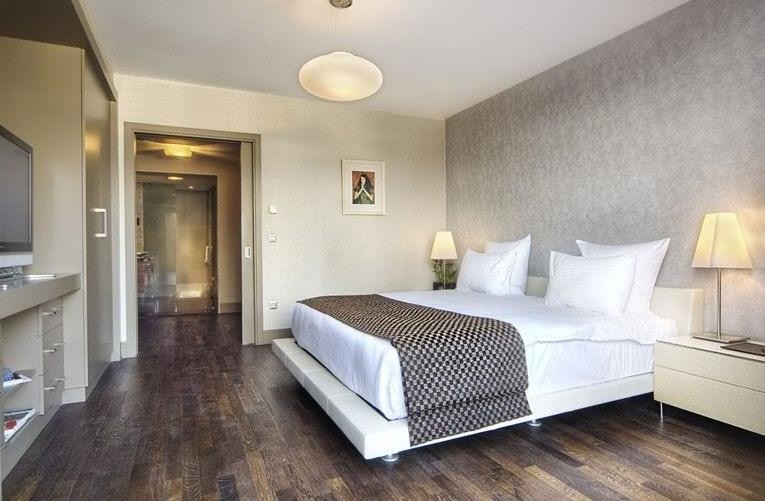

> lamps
[431,231,459,288]
[299,0,383,102]
[691,212,754,343]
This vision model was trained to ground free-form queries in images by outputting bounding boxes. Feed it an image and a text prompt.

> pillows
[483,234,531,294]
[456,249,517,295]
[544,248,636,313]
[576,238,671,311]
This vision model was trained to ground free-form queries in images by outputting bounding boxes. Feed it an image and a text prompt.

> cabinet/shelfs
[0,37,113,404]
[1,273,81,486]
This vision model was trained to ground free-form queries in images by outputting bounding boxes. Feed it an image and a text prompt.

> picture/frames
[342,159,385,215]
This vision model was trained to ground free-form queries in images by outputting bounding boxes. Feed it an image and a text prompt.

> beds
[271,275,707,461]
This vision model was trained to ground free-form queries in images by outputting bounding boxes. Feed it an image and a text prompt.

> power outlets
[270,301,277,309]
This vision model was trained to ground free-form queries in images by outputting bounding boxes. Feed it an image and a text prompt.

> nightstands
[654,335,765,436]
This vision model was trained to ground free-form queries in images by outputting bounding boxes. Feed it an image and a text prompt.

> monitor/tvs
[0,125,34,289]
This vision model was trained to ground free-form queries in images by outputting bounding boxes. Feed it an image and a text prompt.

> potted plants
[433,259,458,290]
